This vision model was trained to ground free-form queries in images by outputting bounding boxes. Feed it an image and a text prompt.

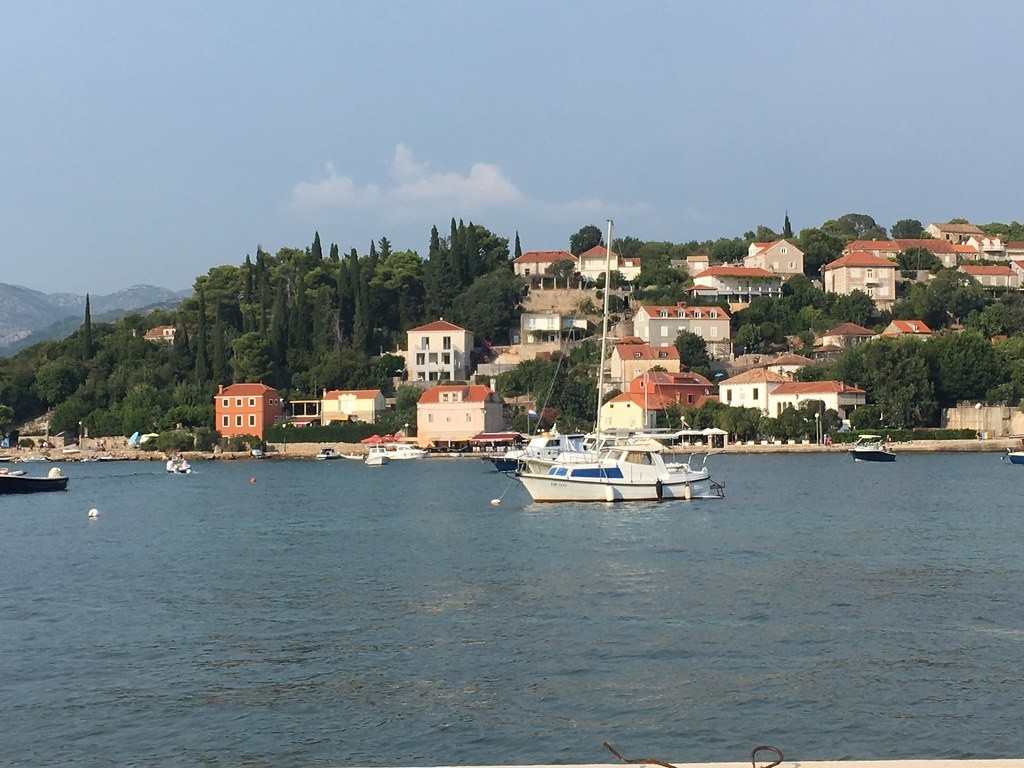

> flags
[529,409,538,418]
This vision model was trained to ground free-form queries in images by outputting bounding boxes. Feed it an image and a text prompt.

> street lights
[680,416,685,449]
[815,413,820,446]
[405,423,409,438]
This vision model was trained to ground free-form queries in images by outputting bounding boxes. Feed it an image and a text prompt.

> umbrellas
[128,432,138,445]
[676,428,702,442]
[700,428,729,447]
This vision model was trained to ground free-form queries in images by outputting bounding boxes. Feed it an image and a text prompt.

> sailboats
[503,211,729,503]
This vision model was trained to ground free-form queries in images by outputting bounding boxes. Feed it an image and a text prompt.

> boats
[480,434,585,472]
[515,431,691,473]
[364,444,390,466]
[21,456,53,463]
[1008,435,1024,464]
[169,463,192,474]
[316,447,340,461]
[98,455,130,461]
[383,444,429,460]
[62,450,82,454]
[850,434,896,462]
[251,449,269,460]
[0,454,13,462]
[361,434,403,444]
[0,466,70,495]
[337,451,365,460]
[81,458,98,462]
